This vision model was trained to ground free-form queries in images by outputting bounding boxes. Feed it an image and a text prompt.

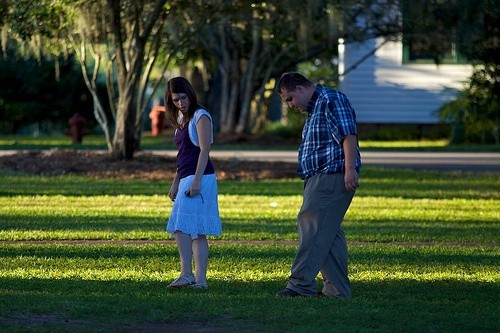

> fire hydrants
[64,113,93,145]
[148,105,167,137]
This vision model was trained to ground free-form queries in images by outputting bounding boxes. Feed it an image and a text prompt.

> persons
[275,72,362,301]
[165,77,222,292]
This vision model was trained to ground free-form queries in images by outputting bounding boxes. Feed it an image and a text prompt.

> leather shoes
[276,289,301,298]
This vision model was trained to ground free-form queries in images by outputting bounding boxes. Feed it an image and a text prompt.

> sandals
[167,274,208,288]
